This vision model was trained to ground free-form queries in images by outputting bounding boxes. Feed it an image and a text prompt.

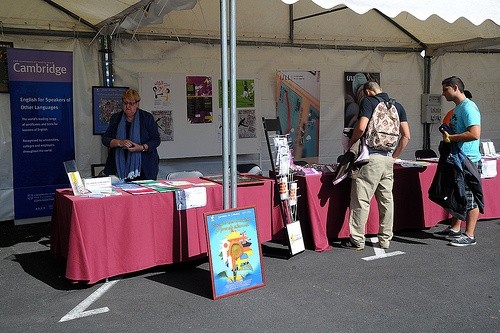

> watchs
[445,135,450,143]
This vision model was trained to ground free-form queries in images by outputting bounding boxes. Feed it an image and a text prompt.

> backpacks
[362,96,401,153]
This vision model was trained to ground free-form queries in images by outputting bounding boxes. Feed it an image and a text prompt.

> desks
[51,173,290,286]
[269,154,500,253]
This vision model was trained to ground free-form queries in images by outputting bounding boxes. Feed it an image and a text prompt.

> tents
[0,0,500,210]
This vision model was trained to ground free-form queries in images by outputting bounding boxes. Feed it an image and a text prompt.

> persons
[102,89,161,182]
[428,76,485,247]
[341,81,410,251]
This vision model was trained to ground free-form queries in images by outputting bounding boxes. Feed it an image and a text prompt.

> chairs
[414,149,438,159]
[167,170,203,179]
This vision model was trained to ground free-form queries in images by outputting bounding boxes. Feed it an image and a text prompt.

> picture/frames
[92,86,130,135]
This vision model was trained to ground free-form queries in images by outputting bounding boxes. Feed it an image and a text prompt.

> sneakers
[340,237,364,250]
[448,232,477,247]
[433,225,462,238]
[377,241,389,249]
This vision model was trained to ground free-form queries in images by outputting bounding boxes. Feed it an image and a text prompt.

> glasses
[122,100,138,105]
